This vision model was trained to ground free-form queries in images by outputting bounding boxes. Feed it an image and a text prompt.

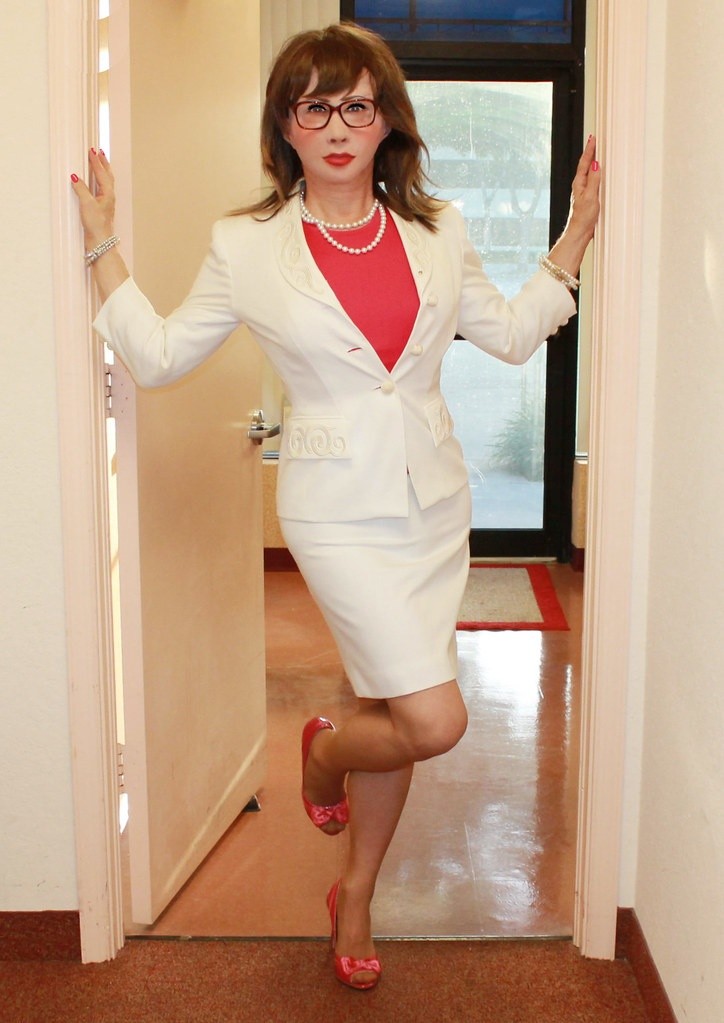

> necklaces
[301,187,386,254]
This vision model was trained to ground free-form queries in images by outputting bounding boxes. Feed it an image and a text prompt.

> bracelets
[85,236,119,267]
[537,256,581,290]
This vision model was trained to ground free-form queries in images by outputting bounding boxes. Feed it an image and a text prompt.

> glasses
[288,95,382,130]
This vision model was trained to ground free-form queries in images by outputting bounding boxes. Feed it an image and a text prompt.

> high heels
[326,878,382,990]
[301,716,349,836]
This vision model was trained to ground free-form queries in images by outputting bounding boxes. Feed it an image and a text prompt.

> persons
[72,23,600,987]
